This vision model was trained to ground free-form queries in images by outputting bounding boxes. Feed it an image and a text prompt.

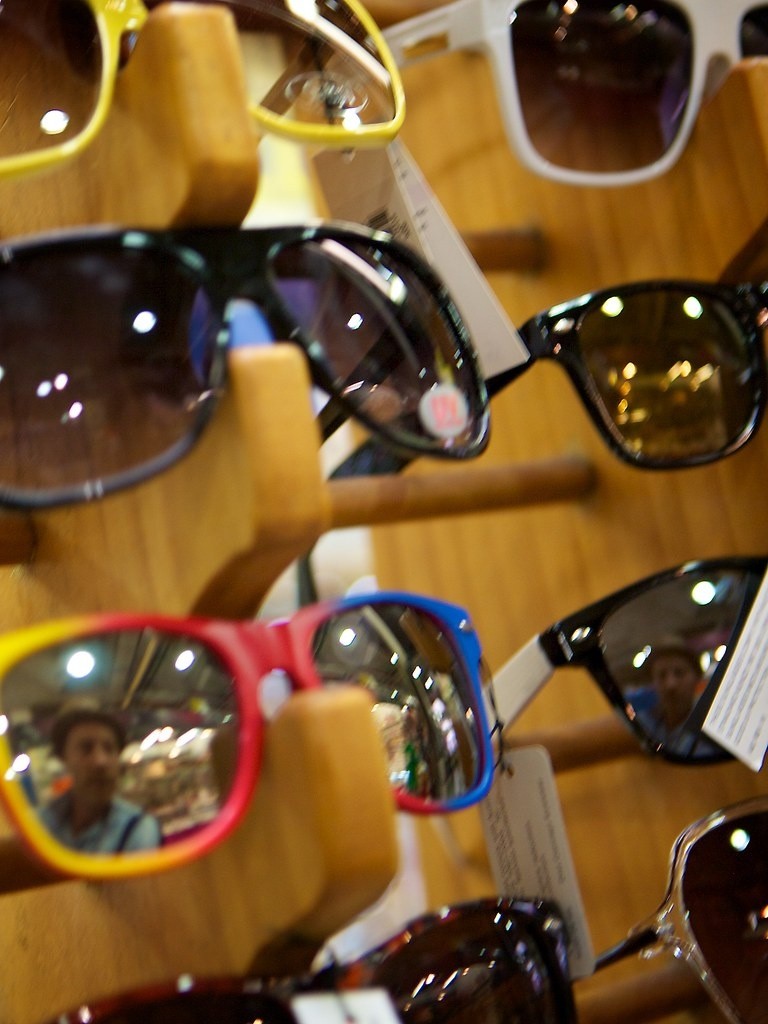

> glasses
[368,1,767,185]
[579,795,767,1024]
[0,590,499,880]
[480,224,768,472]
[42,896,582,1024]
[0,1,406,181]
[483,555,764,769]
[3,221,490,506]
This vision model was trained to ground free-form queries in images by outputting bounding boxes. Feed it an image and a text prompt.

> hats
[45,697,127,756]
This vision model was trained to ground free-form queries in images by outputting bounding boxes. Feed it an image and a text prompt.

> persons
[36,701,160,853]
[635,646,714,755]
